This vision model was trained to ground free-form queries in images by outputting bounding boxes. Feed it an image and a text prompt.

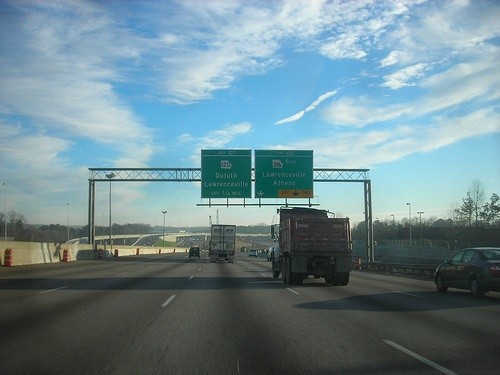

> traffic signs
[200,148,252,198]
[254,150,314,198]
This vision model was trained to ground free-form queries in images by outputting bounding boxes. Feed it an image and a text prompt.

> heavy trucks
[270,206,353,285]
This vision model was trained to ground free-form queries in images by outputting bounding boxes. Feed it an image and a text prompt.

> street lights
[417,211,424,247]
[161,210,167,248]
[3,182,9,241]
[390,214,395,248]
[406,202,412,246]
[105,172,116,245]
[66,202,70,242]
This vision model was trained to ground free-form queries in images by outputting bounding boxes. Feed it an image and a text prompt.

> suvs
[189,247,201,258]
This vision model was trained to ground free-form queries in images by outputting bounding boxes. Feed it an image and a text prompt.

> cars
[266,247,272,261]
[433,247,500,297]
[249,248,258,257]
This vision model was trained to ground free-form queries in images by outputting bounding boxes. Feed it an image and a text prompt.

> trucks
[208,224,237,264]
